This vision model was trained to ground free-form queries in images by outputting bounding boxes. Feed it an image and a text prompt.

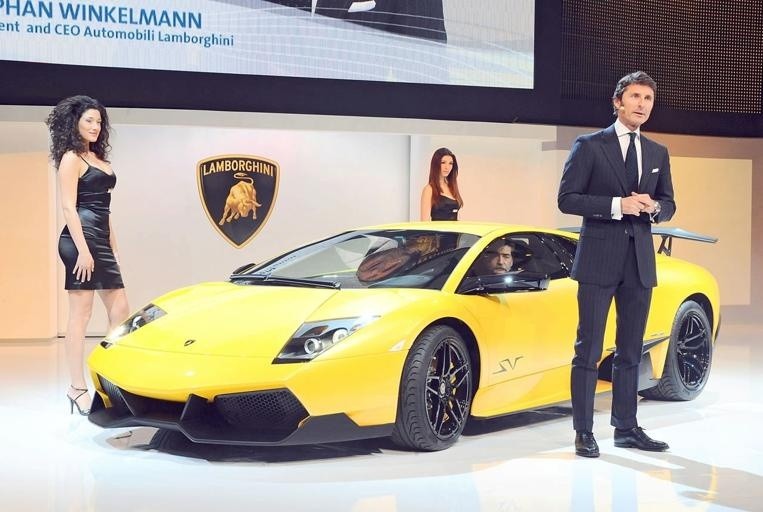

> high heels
[67,385,93,416]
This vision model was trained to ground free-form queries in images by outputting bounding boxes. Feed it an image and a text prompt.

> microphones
[614,106,625,113]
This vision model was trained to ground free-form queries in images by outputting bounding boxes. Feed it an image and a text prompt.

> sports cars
[82,221,726,456]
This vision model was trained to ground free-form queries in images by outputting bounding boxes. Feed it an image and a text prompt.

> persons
[419,147,463,250]
[556,69,679,457]
[46,95,131,418]
[480,239,516,276]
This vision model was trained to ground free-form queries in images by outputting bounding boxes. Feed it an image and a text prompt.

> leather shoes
[575,431,599,457]
[614,426,669,452]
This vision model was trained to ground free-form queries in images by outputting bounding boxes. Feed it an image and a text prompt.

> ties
[625,133,638,195]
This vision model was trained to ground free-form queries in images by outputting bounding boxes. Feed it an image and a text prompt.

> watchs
[654,200,661,215]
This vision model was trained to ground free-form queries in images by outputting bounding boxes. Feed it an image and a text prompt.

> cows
[219,172,262,226]
